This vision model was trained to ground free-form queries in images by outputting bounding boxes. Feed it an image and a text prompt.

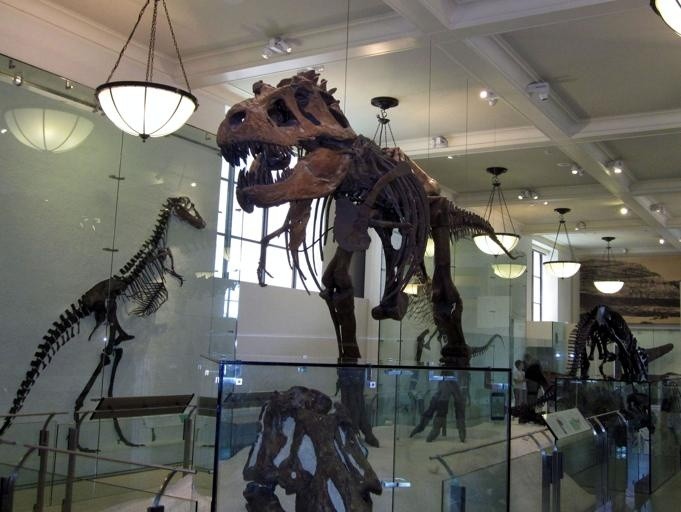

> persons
[522,351,549,409]
[513,354,528,408]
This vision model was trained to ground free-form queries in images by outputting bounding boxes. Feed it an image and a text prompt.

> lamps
[593,237,624,294]
[570,163,585,176]
[261,37,291,59]
[543,207,581,279]
[492,263,526,279]
[472,166,520,258]
[518,190,538,203]
[1,104,95,153]
[605,156,624,174]
[96,0,198,143]
[649,202,665,215]
